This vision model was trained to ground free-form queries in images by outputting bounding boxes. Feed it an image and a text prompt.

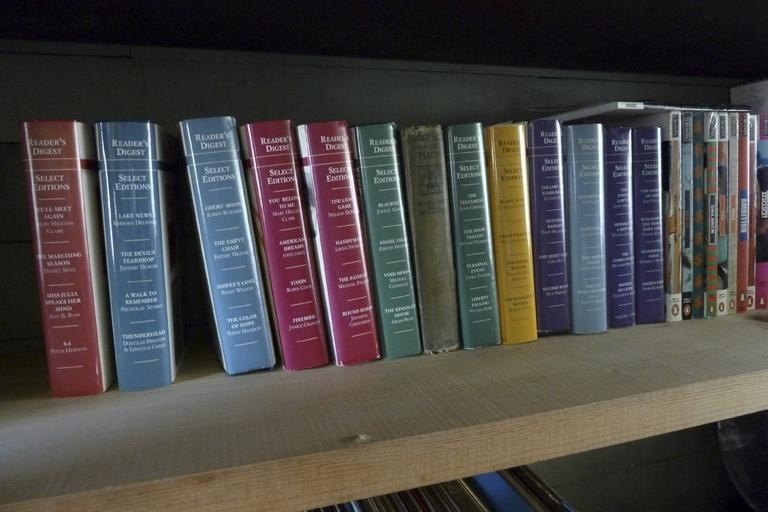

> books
[21,121,115,396]
[178,80,767,375]
[96,121,195,392]
